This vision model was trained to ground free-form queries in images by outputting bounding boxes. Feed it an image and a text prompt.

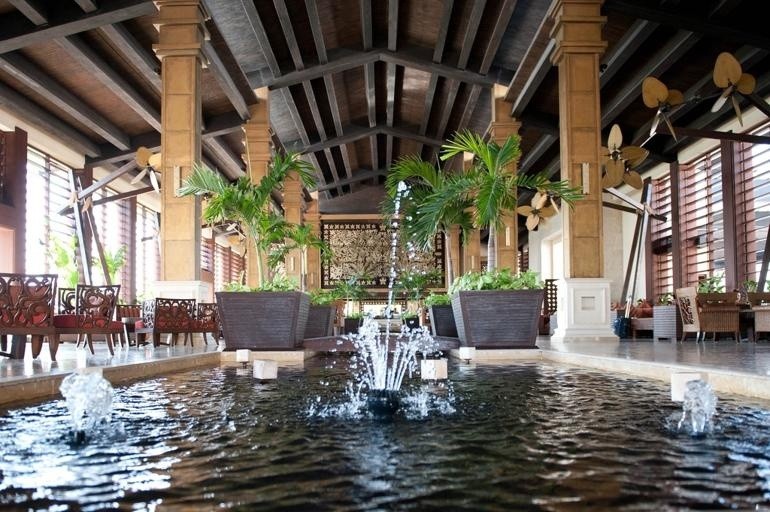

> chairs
[610,286,770,344]
[0,273,225,361]
